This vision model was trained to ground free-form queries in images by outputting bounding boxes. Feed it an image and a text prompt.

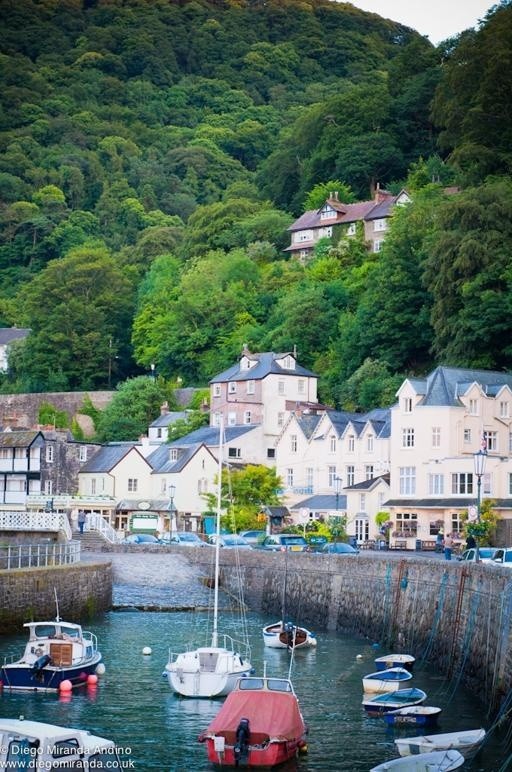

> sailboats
[160,411,254,696]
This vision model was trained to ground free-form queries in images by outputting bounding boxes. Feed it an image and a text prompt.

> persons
[442,534,453,560]
[286,622,296,649]
[466,535,475,549]
[234,719,250,772]
[78,509,87,534]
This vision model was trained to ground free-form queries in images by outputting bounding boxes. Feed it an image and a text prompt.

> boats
[0,621,104,695]
[0,718,122,772]
[360,654,486,771]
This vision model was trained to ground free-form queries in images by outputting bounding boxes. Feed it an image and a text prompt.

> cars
[125,529,359,557]
[455,547,512,567]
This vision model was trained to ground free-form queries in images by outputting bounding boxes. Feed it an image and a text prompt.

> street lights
[168,481,176,544]
[474,448,487,563]
[199,676,305,765]
[151,364,155,377]
[263,621,317,649]
